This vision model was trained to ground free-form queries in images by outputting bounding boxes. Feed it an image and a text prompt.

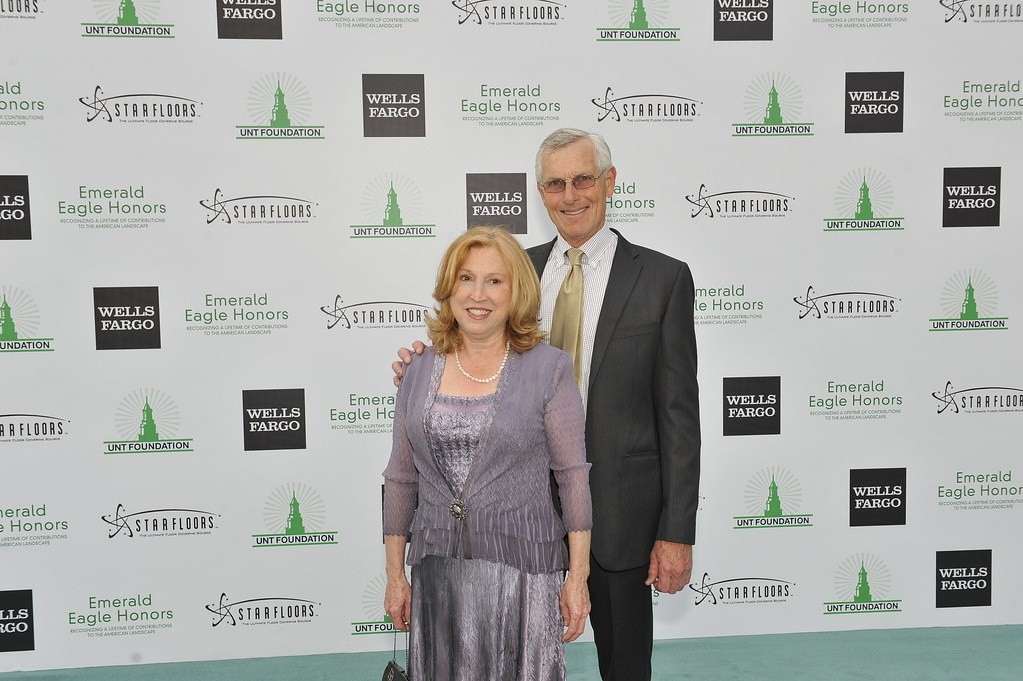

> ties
[549,249,583,394]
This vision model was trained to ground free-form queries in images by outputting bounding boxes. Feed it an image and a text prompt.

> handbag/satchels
[381,662,411,681]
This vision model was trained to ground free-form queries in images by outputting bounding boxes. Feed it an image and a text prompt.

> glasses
[539,171,604,194]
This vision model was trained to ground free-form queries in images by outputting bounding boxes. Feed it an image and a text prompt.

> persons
[391,127,705,681]
[381,224,596,681]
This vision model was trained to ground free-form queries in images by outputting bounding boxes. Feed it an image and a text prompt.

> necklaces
[454,330,511,383]
